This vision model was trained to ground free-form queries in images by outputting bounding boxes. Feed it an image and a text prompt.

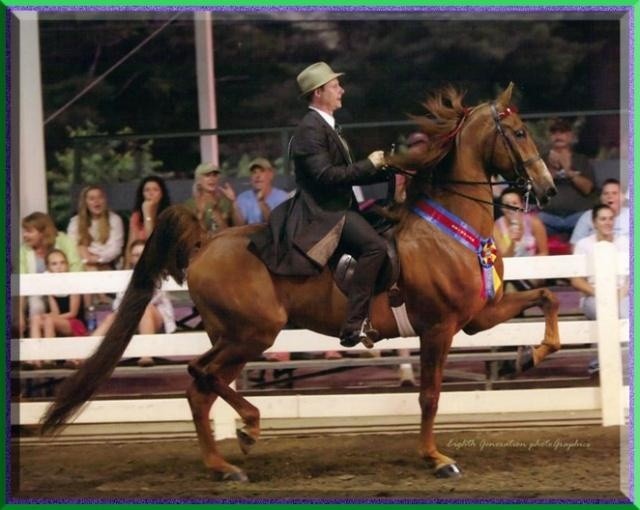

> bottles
[87,306,96,332]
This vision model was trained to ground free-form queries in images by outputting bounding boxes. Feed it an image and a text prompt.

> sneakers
[340,326,379,347]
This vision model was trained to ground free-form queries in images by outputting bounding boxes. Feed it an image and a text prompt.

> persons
[20,111,630,388]
[281,58,405,349]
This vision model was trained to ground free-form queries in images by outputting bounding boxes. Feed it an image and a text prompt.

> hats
[194,164,222,176]
[295,61,346,101]
[248,157,271,171]
[407,132,430,145]
[549,119,570,132]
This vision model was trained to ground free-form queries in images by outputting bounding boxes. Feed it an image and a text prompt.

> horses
[35,81,560,484]
